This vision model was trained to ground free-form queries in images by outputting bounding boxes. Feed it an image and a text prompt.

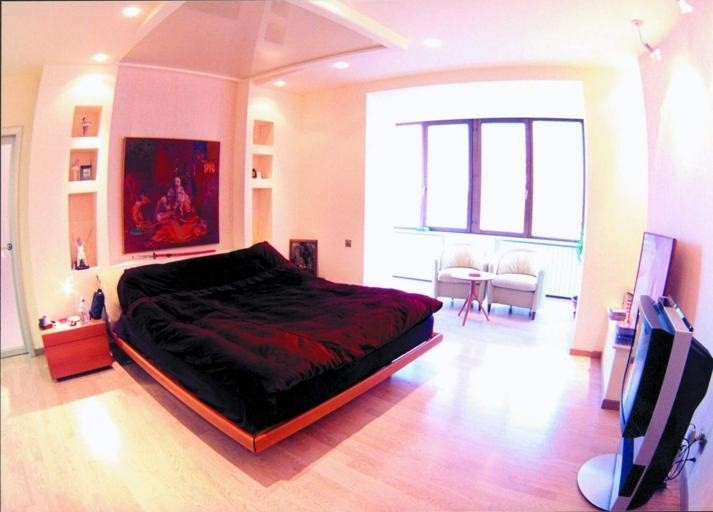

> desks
[451,270,498,326]
[576,453,616,511]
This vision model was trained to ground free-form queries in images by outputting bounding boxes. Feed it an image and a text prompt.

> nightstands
[40,315,114,380]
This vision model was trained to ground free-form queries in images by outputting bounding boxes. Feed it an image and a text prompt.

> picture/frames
[289,239,318,277]
[119,137,223,254]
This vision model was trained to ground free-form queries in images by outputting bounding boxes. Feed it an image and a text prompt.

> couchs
[433,246,487,312]
[485,254,544,319]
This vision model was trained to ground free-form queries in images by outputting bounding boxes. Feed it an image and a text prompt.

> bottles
[80,298,90,326]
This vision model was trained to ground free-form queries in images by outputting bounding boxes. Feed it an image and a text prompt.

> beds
[99,241,443,456]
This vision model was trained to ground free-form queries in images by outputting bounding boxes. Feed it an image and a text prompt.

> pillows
[444,245,481,271]
[495,249,539,278]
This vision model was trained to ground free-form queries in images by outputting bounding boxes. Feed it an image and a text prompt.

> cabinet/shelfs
[600,306,632,410]
[244,115,276,249]
[64,103,102,275]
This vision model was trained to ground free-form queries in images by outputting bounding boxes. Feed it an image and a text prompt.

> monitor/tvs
[606,293,713,512]
